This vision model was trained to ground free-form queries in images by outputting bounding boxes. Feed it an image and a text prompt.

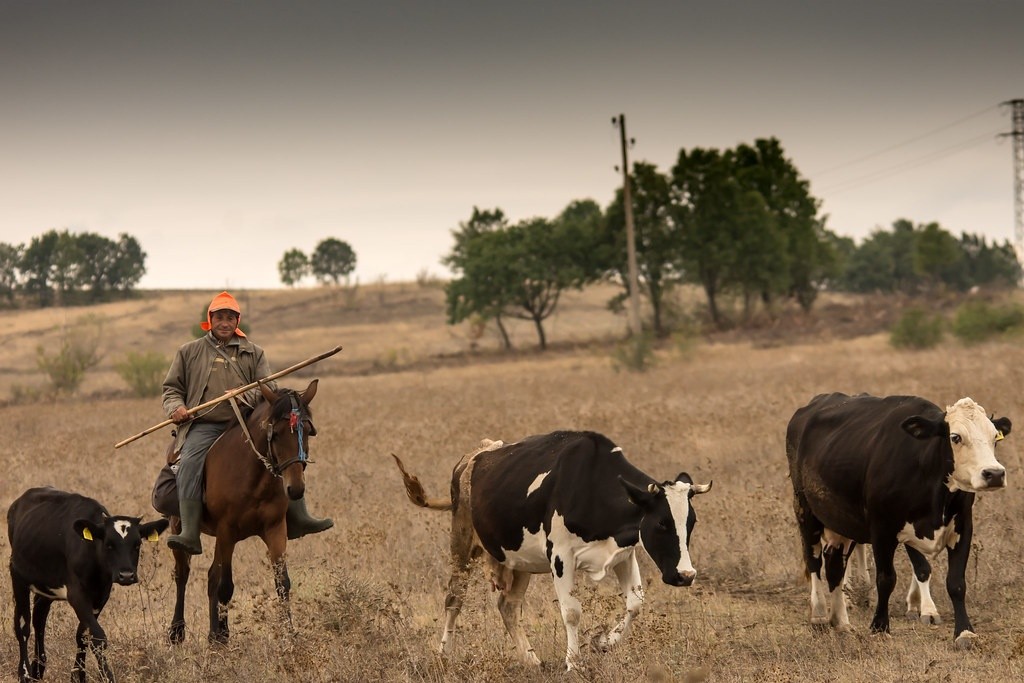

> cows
[786,391,1012,649]
[6,487,170,683]
[391,430,712,670]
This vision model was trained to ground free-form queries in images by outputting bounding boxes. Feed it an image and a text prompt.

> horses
[167,379,319,647]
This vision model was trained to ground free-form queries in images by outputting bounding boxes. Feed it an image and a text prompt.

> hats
[200,291,246,337]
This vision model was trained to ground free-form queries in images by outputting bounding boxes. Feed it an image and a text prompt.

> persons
[162,292,334,555]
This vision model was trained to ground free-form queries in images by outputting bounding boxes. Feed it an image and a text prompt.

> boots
[287,497,334,540]
[167,498,202,555]
